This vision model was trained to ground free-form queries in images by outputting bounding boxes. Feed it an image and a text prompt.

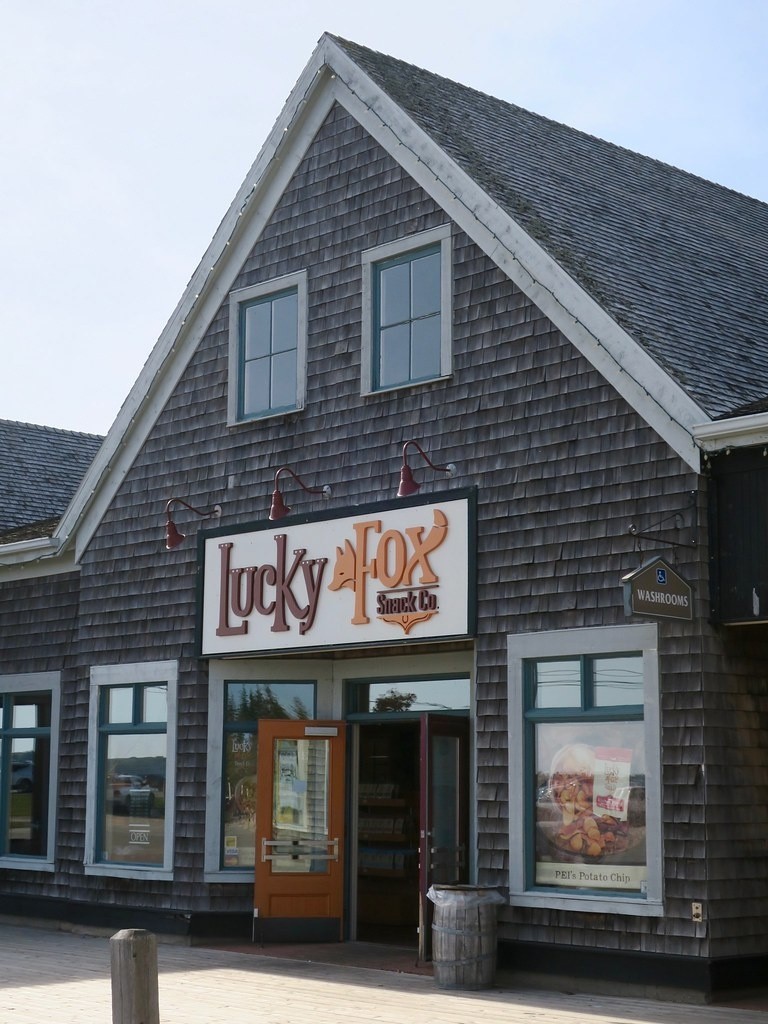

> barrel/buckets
[430,883,502,989]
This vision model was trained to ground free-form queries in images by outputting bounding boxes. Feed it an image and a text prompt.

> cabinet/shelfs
[357,798,406,877]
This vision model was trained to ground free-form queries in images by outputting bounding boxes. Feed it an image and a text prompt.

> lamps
[163,498,223,550]
[268,468,331,522]
[398,441,456,496]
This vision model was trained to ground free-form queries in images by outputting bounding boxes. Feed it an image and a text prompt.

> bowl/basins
[536,787,646,863]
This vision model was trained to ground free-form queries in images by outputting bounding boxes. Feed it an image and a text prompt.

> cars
[107,774,155,812]
[536,777,646,863]
[11,761,37,793]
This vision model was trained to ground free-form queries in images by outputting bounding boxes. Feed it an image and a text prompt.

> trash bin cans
[426,883,507,990]
